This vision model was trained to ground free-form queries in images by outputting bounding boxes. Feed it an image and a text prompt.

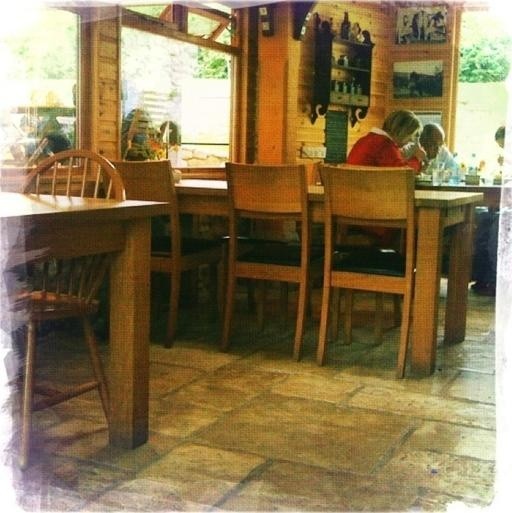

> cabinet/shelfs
[311,11,376,125]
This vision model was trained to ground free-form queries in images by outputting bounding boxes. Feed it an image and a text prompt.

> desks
[176,178,485,377]
[415,173,501,208]
[2,178,170,450]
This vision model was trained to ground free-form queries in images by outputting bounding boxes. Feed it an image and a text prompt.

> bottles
[417,153,478,186]
[341,9,351,38]
[331,79,363,94]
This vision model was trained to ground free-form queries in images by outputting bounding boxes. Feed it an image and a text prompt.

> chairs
[6,148,127,468]
[316,161,416,380]
[107,159,218,349]
[220,161,341,363]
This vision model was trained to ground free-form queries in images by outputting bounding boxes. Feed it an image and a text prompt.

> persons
[472,126,505,296]
[399,123,460,174]
[346,107,428,241]
